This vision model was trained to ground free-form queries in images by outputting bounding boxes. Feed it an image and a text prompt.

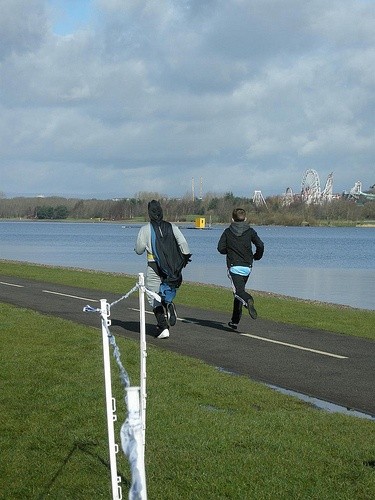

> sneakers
[247,298,257,319]
[228,322,237,329]
[166,302,177,326]
[157,328,170,339]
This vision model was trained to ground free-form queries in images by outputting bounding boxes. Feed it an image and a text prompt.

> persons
[134,200,190,338]
[217,208,264,329]
